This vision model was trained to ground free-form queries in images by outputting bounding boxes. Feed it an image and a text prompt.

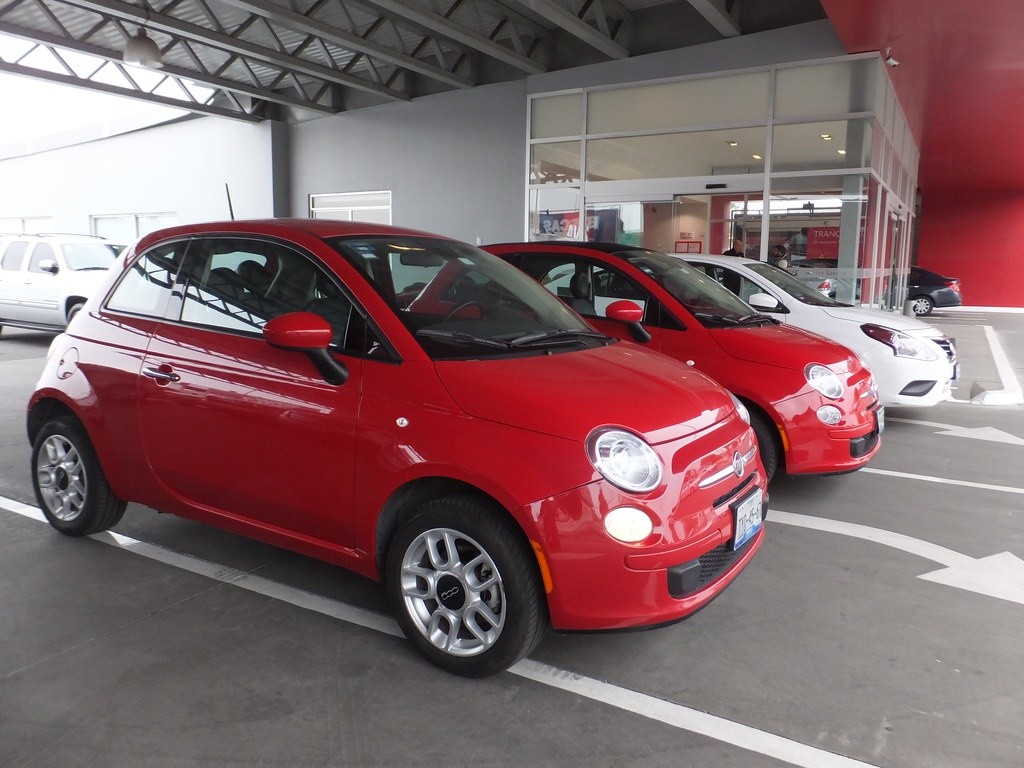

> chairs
[305,244,370,351]
[205,267,244,305]
[620,261,676,328]
[570,272,596,316]
[235,260,271,300]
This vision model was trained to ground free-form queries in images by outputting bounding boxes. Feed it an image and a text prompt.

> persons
[722,240,744,297]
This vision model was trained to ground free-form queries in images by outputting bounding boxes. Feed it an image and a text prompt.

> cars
[542,253,959,408]
[907,264,962,317]
[405,239,883,485]
[0,231,129,338]
[776,265,836,298]
[789,259,863,300]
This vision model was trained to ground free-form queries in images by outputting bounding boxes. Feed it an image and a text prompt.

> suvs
[25,220,769,678]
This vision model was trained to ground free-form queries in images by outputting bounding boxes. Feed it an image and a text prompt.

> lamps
[122,0,163,69]
